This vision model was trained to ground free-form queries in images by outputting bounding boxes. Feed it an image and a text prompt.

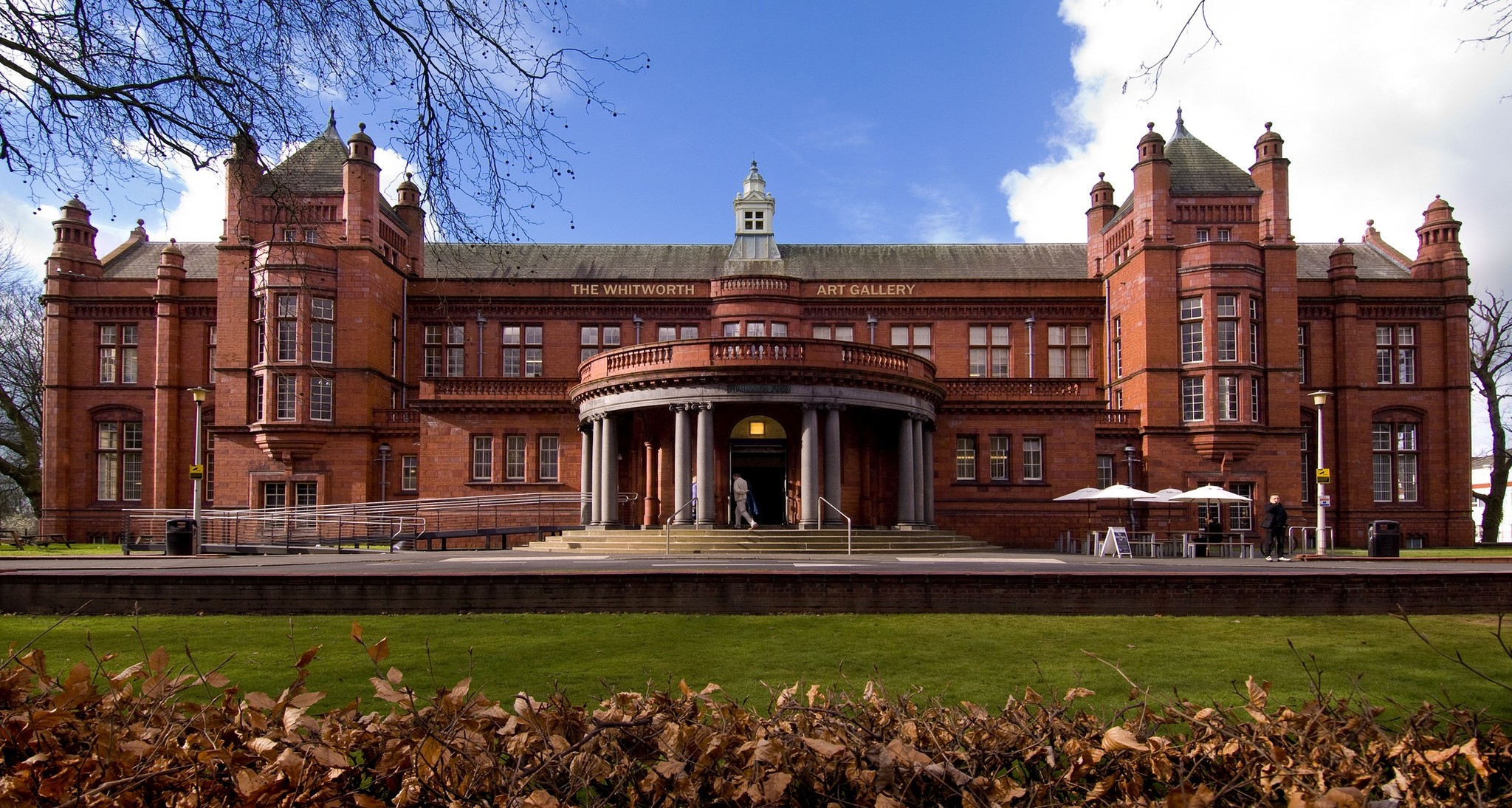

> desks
[15,533,73,551]
[0,530,25,551]
[1060,529,1245,558]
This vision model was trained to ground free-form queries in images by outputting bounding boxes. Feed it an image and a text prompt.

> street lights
[1307,388,1335,556]
[186,384,213,554]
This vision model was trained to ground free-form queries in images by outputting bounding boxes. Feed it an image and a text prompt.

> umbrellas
[1052,484,1106,554]
[1094,481,1163,543]
[1130,485,1189,555]
[1169,483,1254,556]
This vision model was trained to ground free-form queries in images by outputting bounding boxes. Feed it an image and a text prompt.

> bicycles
[1260,522,1298,555]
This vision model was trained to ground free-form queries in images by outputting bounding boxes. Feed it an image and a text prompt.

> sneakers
[1265,556,1273,562]
[731,525,742,529]
[748,524,759,530]
[1277,556,1291,562]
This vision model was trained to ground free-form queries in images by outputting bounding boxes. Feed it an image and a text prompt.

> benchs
[9,540,75,551]
[1054,537,1254,560]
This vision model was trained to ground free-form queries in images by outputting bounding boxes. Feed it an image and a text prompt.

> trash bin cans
[165,517,198,555]
[1366,520,1400,557]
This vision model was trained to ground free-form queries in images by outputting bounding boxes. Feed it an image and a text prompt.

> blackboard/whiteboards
[1103,526,1132,554]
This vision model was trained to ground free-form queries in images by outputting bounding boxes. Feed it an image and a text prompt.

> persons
[1260,492,1292,562]
[1197,516,1223,557]
[691,476,697,518]
[731,473,759,530]
